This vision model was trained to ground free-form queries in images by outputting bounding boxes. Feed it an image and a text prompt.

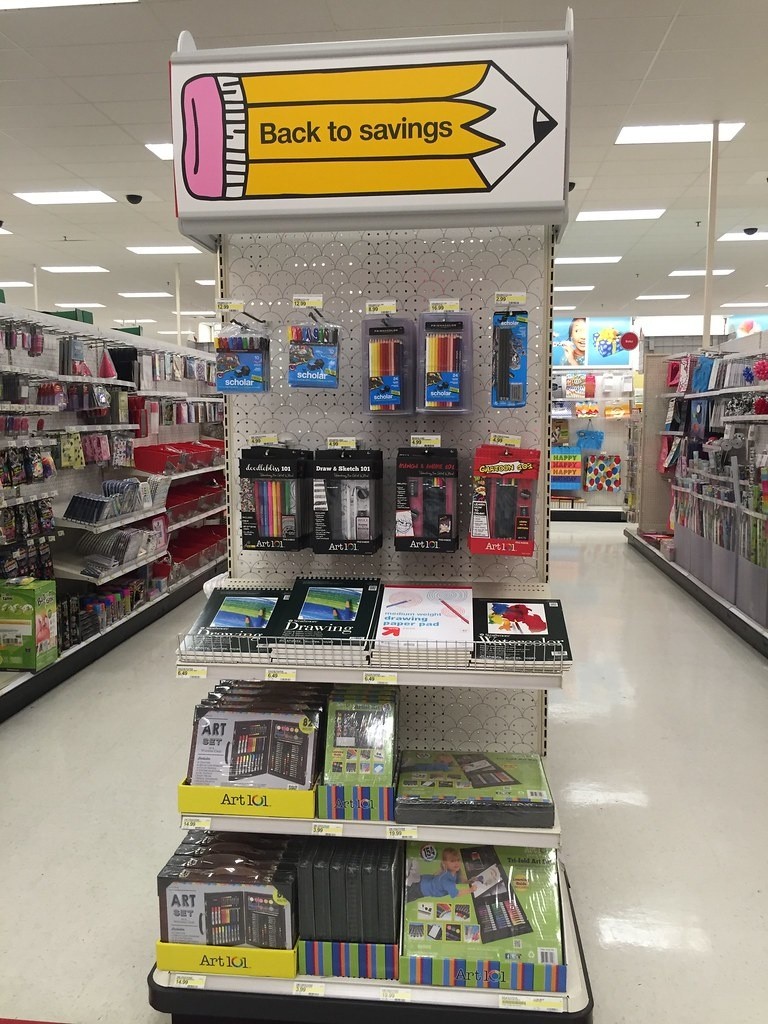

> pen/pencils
[437,597,469,623]
[367,338,404,411]
[425,333,467,408]
[498,329,511,402]
[387,599,413,608]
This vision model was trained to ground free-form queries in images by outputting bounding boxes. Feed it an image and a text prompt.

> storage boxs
[189,480,224,504]
[168,441,213,466]
[395,447,458,553]
[155,937,300,979]
[660,539,675,562]
[174,483,215,513]
[133,445,194,475]
[311,449,378,555]
[296,938,399,980]
[166,488,198,521]
[177,769,323,819]
[199,439,225,466]
[316,750,403,821]
[470,448,534,557]
[0,579,58,674]
[399,841,568,994]
[154,526,227,586]
[239,449,302,552]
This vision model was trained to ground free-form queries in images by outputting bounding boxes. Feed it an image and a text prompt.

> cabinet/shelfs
[147,654,594,1024]
[550,363,632,523]
[633,328,768,658]
[0,303,228,723]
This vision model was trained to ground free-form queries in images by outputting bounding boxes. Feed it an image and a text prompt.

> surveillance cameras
[744,228,758,236]
[126,195,142,204]
[569,182,575,192]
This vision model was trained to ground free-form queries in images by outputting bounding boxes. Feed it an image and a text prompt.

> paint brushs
[214,337,269,351]
[289,326,338,345]
[254,481,286,537]
